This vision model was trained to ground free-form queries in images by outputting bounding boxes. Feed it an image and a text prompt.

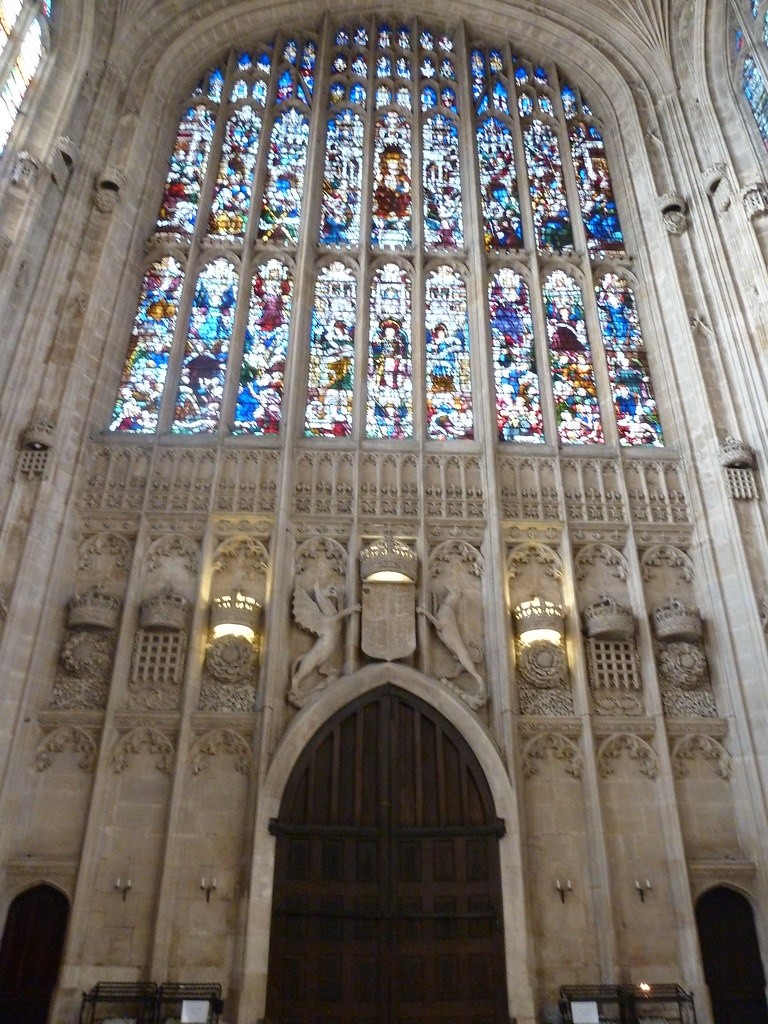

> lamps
[556,878,572,903]
[635,880,651,903]
[114,878,132,902]
[200,878,217,903]
